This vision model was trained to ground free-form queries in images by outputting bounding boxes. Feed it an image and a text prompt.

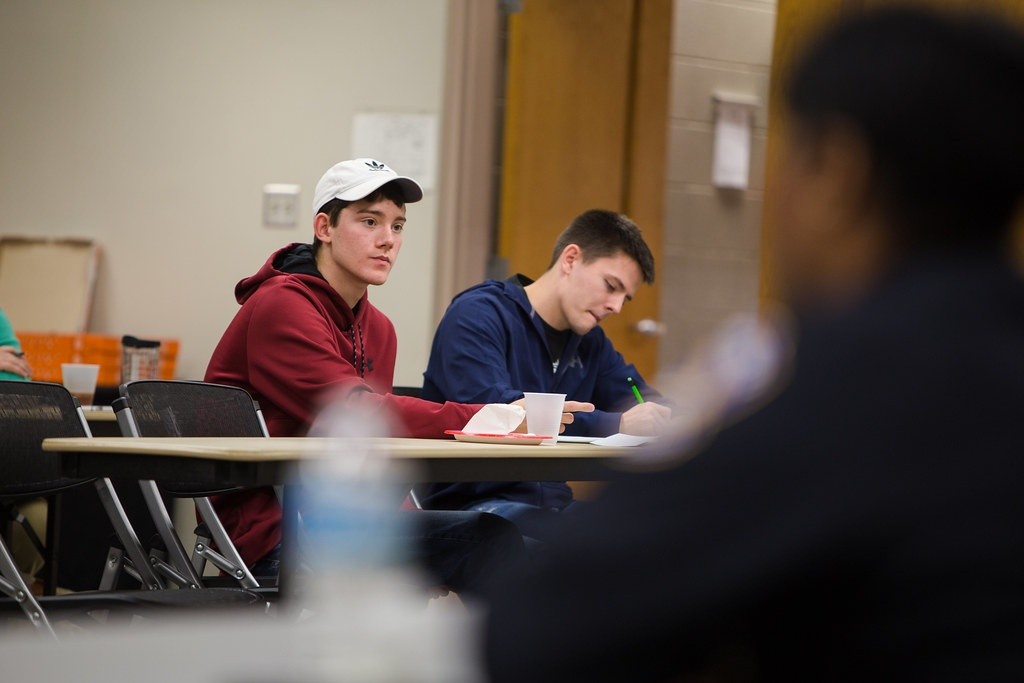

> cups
[60,362,100,410]
[120,334,160,386]
[523,391,567,447]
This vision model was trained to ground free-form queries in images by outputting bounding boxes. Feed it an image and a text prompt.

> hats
[311,158,423,216]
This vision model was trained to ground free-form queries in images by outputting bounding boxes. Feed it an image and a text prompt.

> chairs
[112,379,311,631]
[0,380,264,645]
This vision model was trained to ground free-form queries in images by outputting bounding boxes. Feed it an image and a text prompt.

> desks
[42,437,679,621]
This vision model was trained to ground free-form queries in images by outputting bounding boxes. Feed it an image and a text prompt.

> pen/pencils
[15,352,25,357]
[627,376,644,405]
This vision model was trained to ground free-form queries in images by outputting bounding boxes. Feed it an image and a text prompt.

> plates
[445,428,547,447]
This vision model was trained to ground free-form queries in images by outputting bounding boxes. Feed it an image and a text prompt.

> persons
[0,308,34,383]
[479,0,1024,683]
[192,160,527,603]
[412,209,692,546]
